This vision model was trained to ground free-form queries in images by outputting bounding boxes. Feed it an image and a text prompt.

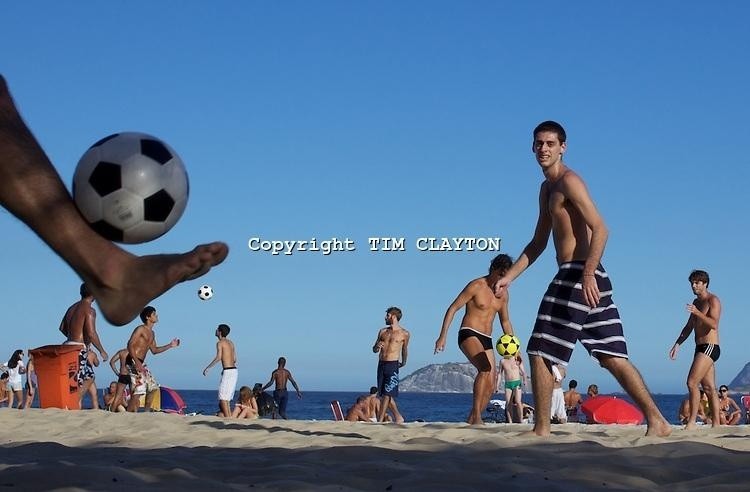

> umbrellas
[581,395,644,425]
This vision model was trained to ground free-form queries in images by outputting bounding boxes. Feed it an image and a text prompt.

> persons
[362,387,381,422]
[678,390,707,426]
[0,371,9,403]
[496,357,527,424]
[23,350,37,409]
[434,254,523,426]
[383,412,392,422]
[104,381,128,412]
[670,269,721,431]
[262,357,302,419]
[125,305,180,412]
[231,386,258,419]
[563,380,584,424]
[78,342,99,409]
[346,395,374,422]
[1,350,27,409]
[0,74,229,327]
[373,307,411,423]
[109,340,137,412]
[550,361,567,424]
[490,121,673,440]
[59,282,109,402]
[203,324,238,418]
[719,385,742,425]
[586,385,598,424]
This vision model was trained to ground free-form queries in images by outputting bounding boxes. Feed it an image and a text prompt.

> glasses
[21,353,24,356]
[719,390,727,392]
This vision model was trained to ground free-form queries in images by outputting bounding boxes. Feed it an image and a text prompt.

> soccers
[72,131,191,245]
[495,334,521,360]
[197,284,215,302]
